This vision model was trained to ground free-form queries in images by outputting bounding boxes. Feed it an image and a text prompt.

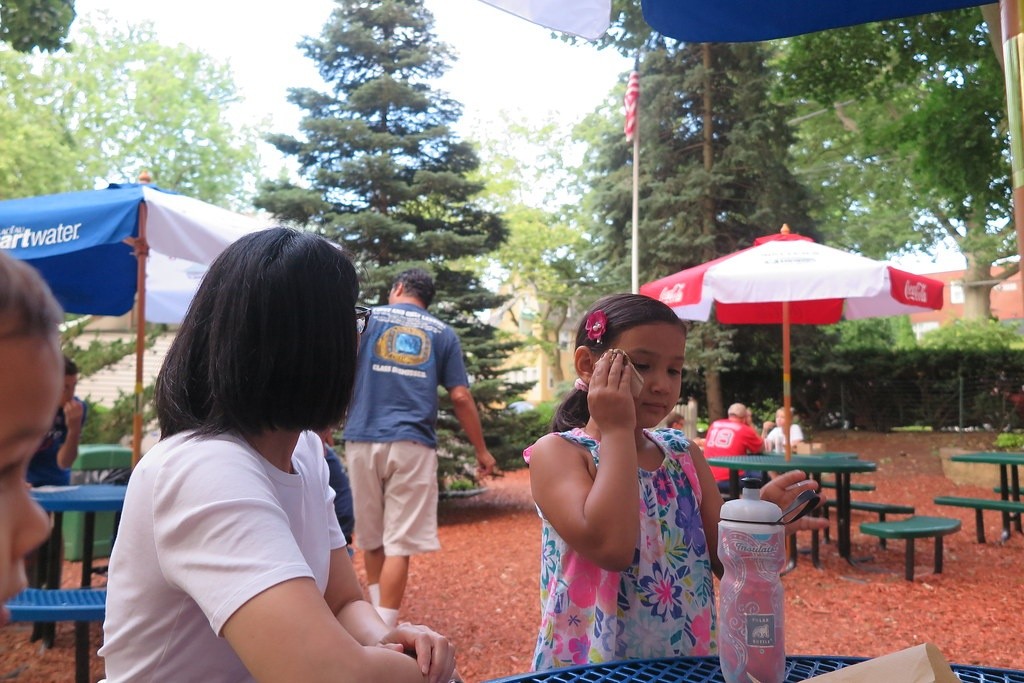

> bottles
[718,476,820,683]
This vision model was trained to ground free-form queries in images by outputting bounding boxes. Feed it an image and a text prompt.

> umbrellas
[0,181,341,471]
[639,223,944,463]
[474,1,1022,286]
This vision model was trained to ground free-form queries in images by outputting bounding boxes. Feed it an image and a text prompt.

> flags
[619,54,635,142]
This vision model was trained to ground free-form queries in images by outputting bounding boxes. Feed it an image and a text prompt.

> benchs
[860,515,962,582]
[826,499,915,551]
[821,479,876,490]
[5,587,107,683]
[933,495,1024,544]
[993,485,1024,495]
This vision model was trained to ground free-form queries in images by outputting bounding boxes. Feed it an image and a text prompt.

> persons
[666,402,805,494]
[523,293,831,673]
[95,227,455,683]
[0,255,83,683]
[339,272,506,630]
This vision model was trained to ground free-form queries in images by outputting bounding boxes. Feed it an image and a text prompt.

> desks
[706,455,877,568]
[27,484,129,589]
[951,451,1024,539]
[483,655,1024,683]
